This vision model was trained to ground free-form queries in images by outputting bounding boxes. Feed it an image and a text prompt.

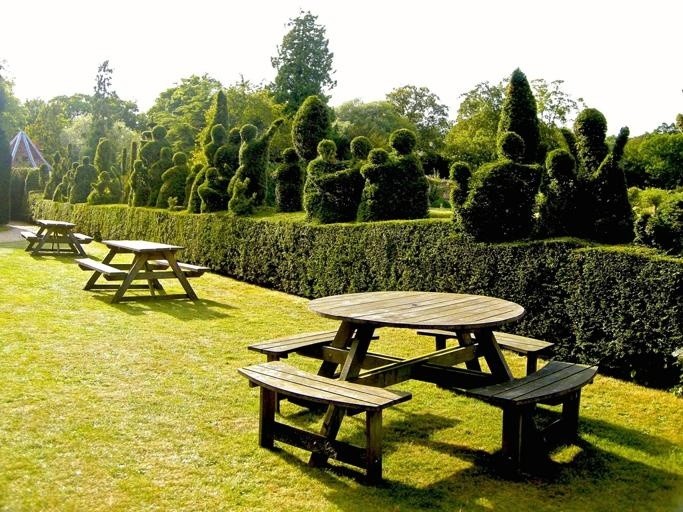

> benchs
[248,329,379,413]
[466,360,600,483]
[236,361,412,485]
[74,257,128,281]
[73,233,94,244]
[21,231,38,242]
[416,329,554,410]
[149,260,211,277]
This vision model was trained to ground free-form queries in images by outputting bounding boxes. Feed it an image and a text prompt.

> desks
[24,220,87,256]
[82,240,199,304]
[307,290,552,469]
